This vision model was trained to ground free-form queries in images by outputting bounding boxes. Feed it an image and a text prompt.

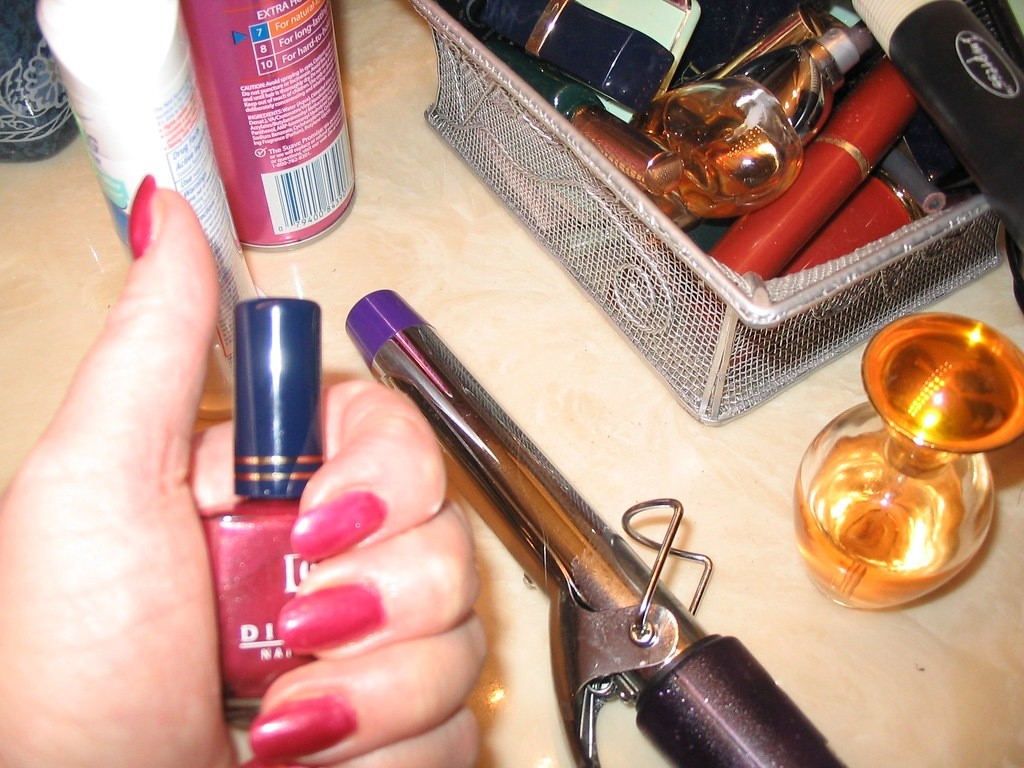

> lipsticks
[694,58,918,283]
[481,0,675,113]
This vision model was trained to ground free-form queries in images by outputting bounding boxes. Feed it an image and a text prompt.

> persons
[0,175,490,768]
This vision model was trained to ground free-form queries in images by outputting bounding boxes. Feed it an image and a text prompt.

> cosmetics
[480,0,1024,612]
[203,296,327,701]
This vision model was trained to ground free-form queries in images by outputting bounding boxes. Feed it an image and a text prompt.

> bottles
[1,0,81,166]
[37,0,263,419]
[794,312,1024,609]
[180,0,356,250]
